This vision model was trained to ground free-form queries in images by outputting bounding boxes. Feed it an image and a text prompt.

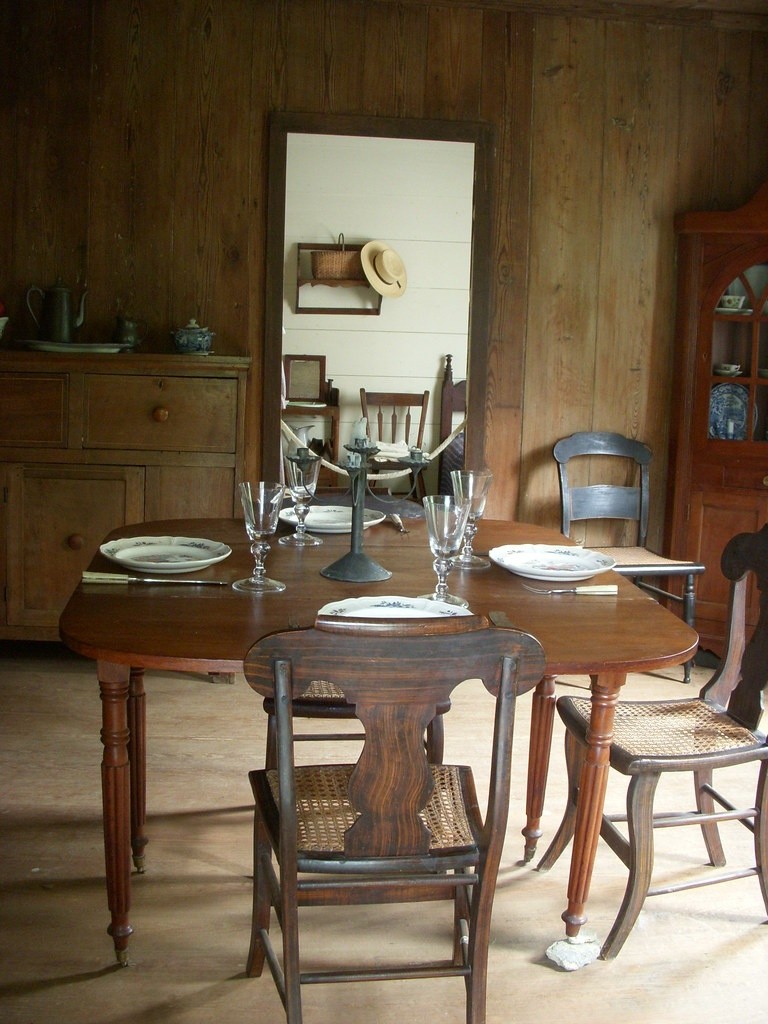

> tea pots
[27,275,88,342]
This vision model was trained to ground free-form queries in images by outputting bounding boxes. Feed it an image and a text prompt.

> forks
[390,513,410,533]
[521,583,618,595]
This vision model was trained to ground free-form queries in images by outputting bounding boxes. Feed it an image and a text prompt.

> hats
[361,240,407,299]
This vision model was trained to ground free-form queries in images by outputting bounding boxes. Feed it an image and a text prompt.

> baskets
[312,233,366,279]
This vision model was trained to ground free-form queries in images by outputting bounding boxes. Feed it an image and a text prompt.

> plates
[17,340,129,353]
[99,535,232,573]
[280,506,387,533]
[714,370,742,377]
[710,383,758,440]
[318,596,474,618]
[489,543,617,581]
[716,308,754,315]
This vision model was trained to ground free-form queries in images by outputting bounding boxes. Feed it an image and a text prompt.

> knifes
[82,570,229,584]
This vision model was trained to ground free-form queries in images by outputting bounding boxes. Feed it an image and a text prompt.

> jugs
[289,424,316,455]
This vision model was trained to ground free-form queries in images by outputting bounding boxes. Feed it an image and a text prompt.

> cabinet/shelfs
[0,350,253,685]
[296,243,382,315]
[660,176,767,658]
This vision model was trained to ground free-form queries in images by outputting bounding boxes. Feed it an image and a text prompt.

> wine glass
[278,456,323,546]
[450,470,491,570]
[417,495,469,608]
[232,482,286,593]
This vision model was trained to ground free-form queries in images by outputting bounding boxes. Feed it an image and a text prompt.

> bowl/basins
[0,317,8,337]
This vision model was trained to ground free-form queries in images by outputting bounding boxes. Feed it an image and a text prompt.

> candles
[356,417,368,438]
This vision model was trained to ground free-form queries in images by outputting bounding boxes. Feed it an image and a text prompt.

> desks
[55,519,697,970]
[283,355,339,487]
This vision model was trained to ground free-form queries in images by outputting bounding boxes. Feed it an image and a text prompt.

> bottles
[411,447,422,462]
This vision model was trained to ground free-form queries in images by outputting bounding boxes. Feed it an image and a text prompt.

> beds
[439,354,466,496]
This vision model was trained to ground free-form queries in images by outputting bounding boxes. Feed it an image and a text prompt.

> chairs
[539,524,767,963]
[263,496,452,848]
[359,388,430,506]
[553,429,705,683]
[243,615,547,1024]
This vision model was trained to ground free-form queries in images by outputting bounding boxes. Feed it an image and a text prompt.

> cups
[114,317,148,353]
[721,364,740,371]
[174,319,213,356]
[721,295,745,308]
